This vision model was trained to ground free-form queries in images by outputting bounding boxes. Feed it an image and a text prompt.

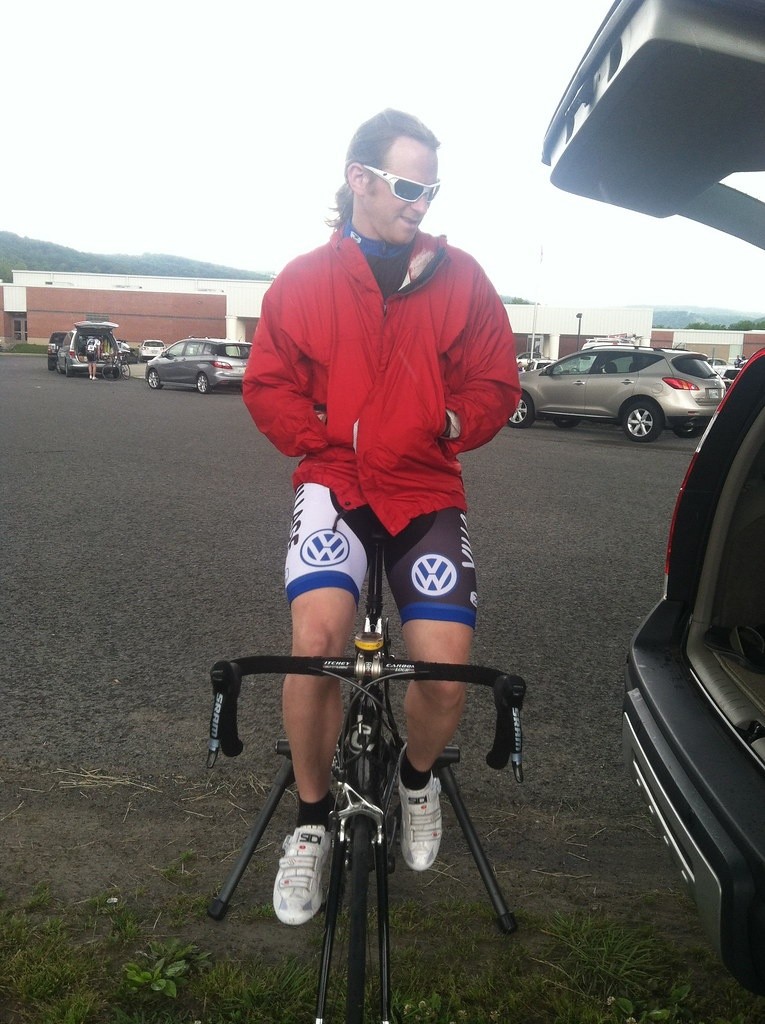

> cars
[515,349,557,372]
[708,356,750,390]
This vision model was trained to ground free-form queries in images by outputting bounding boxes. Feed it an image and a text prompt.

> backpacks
[87,339,98,361]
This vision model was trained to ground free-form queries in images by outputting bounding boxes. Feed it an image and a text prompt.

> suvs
[117,339,135,364]
[54,320,124,378]
[577,333,642,373]
[541,0,764,997]
[47,330,70,372]
[137,339,169,363]
[144,335,253,394]
[505,344,726,443]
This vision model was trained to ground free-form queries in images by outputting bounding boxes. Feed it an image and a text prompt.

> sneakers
[397,743,444,872]
[273,825,330,925]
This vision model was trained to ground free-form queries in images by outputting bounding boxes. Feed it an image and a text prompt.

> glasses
[363,165,441,203]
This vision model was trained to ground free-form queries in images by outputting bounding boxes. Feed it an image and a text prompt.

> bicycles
[101,352,132,382]
[203,528,532,1024]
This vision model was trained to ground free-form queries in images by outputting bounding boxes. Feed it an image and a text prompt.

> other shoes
[90,375,98,380]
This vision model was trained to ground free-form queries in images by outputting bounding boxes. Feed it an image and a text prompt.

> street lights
[576,312,583,351]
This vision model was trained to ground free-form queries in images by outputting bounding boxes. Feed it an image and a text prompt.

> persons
[734,355,746,368]
[86,336,100,381]
[243,108,518,925]
